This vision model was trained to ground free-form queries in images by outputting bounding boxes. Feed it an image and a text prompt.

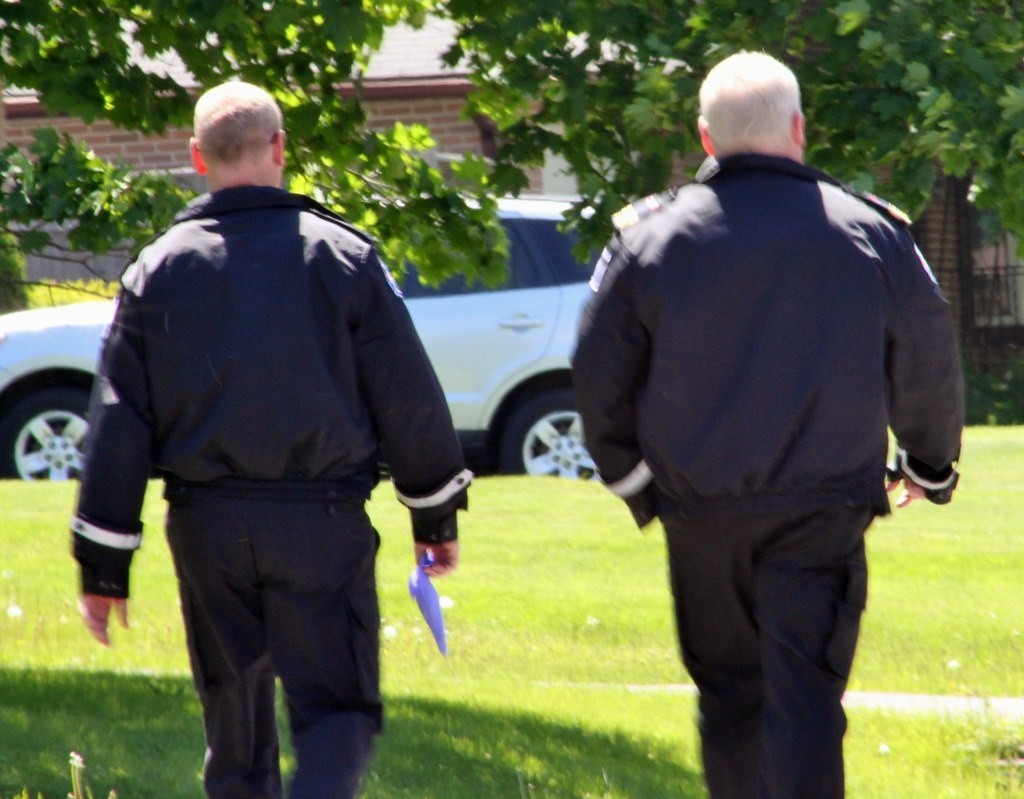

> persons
[70,82,473,799]
[571,52,964,799]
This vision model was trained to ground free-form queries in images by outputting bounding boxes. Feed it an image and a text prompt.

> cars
[0,195,602,483]
